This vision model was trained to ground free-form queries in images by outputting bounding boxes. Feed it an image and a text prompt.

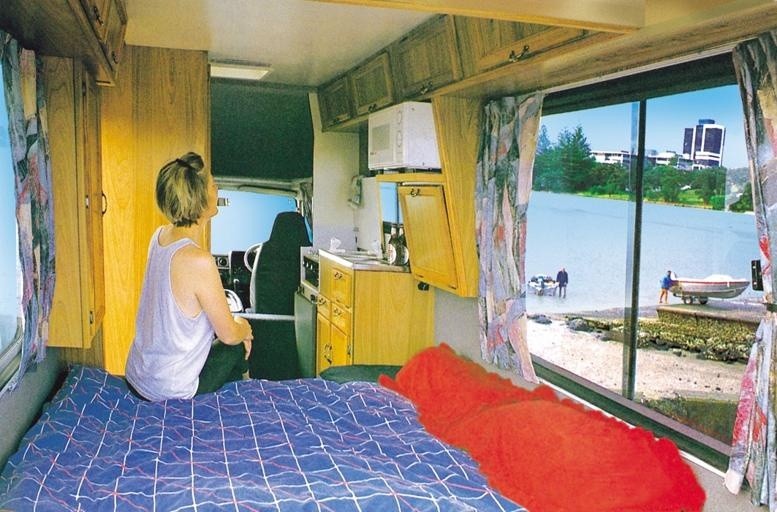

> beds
[2,361,737,512]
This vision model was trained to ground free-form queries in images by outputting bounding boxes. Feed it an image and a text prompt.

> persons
[555,268,568,299]
[656,270,673,303]
[122,151,253,404]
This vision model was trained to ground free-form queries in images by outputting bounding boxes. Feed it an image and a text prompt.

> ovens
[290,285,316,380]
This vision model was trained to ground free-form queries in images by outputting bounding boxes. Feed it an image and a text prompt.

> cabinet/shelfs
[316,12,585,133]
[396,182,480,299]
[315,255,436,377]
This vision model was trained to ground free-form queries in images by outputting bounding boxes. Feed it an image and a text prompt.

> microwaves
[367,100,443,172]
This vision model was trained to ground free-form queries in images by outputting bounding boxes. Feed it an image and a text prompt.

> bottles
[397,225,409,265]
[388,224,401,266]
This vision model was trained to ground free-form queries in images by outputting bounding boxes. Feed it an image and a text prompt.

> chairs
[248,210,313,315]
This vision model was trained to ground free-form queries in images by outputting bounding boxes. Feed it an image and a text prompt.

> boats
[667,272,753,296]
[529,275,559,297]
